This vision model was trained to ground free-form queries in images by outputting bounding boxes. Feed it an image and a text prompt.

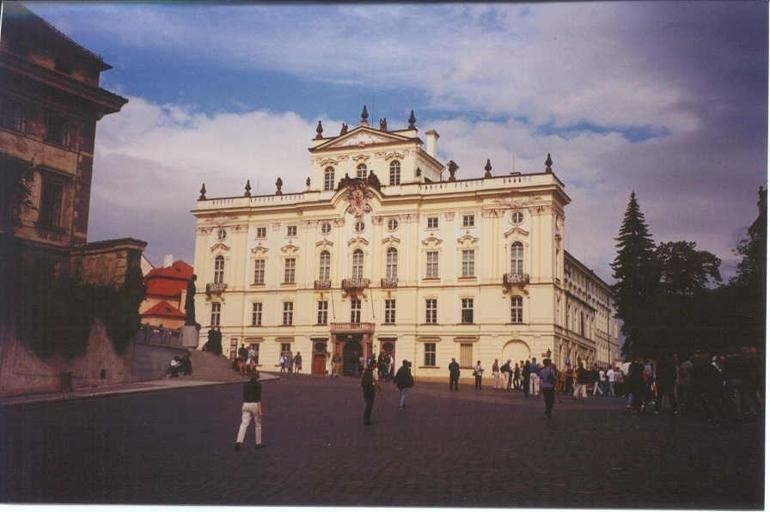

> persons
[184,274,198,322]
[165,355,182,377]
[232,342,257,377]
[331,351,394,384]
[180,352,193,376]
[235,370,266,451]
[279,351,303,375]
[207,324,216,342]
[474,360,482,390]
[392,360,415,410]
[491,343,765,429]
[359,358,382,427]
[217,327,222,354]
[448,357,460,391]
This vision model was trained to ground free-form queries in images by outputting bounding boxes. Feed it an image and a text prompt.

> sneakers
[255,444,265,448]
[236,442,241,451]
[545,410,552,419]
